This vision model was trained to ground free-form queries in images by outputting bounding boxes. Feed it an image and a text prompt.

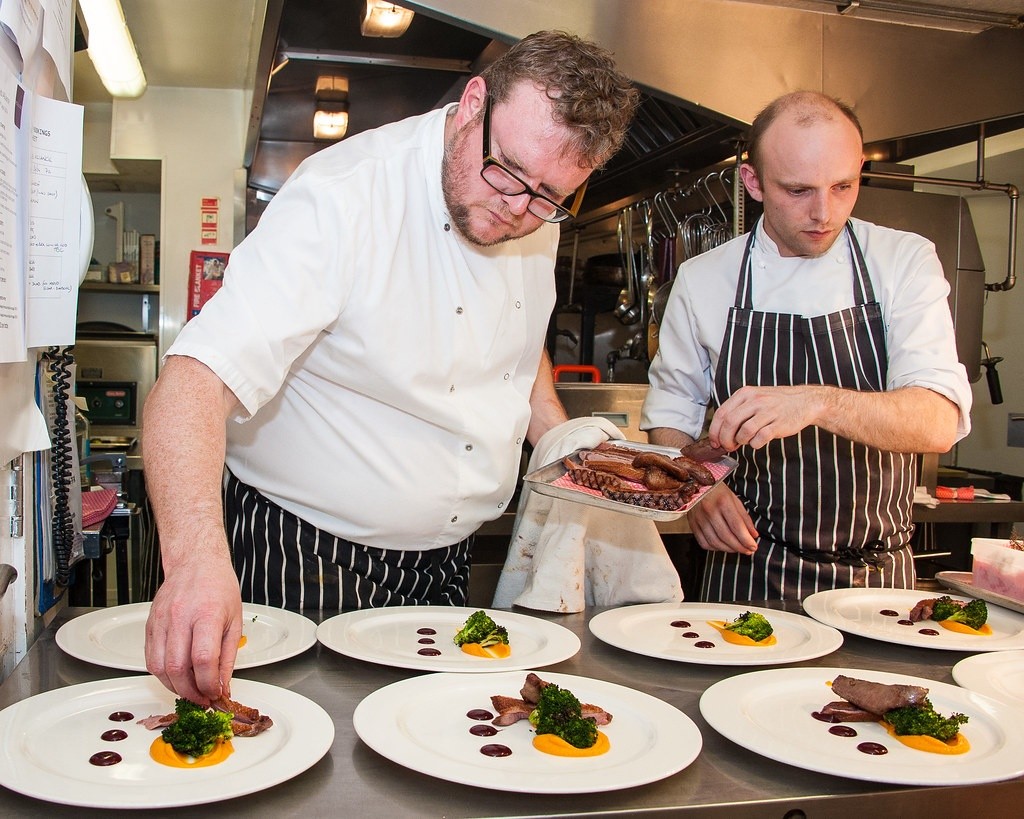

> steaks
[910,597,968,622]
[135,690,273,737]
[819,674,930,722]
[560,432,726,511]
[488,673,613,727]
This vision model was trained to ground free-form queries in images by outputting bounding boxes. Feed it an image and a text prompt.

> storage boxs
[84,257,103,281]
[969,537,1024,602]
[140,233,155,285]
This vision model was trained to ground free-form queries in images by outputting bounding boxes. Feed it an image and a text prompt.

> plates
[0,674,334,809]
[588,601,844,666]
[803,588,1024,651]
[55,600,317,672]
[952,649,1024,718]
[353,670,703,794]
[701,667,1024,786]
[315,606,581,673]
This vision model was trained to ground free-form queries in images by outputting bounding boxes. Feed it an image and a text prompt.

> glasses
[480,92,575,224]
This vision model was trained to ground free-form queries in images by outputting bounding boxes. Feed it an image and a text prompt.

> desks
[471,501,1024,540]
[0,598,1024,819]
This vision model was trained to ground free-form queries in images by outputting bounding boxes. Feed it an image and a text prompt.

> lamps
[79,0,148,99]
[359,0,416,39]
[312,69,351,101]
[312,101,351,139]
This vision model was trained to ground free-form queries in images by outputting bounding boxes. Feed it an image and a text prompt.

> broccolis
[529,683,599,749]
[932,595,987,630]
[883,696,968,741]
[453,610,509,648]
[161,698,235,756]
[724,611,773,642]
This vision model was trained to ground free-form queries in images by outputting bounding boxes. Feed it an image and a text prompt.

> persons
[140,31,639,708]
[637,91,973,603]
